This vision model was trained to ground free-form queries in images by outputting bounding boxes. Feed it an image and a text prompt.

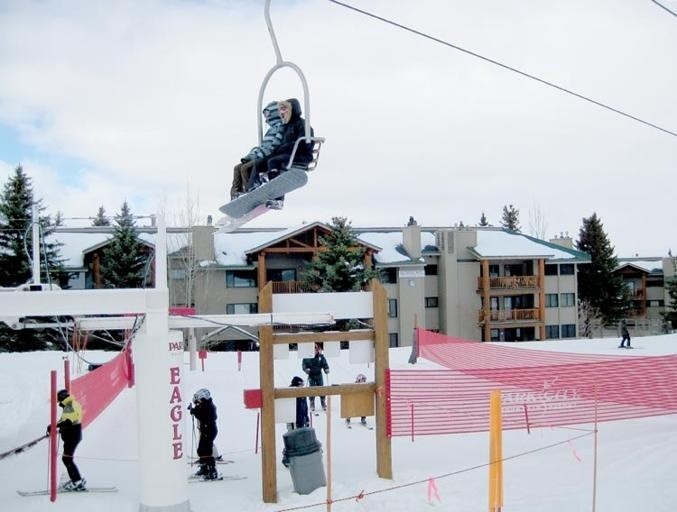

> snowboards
[218,169,308,218]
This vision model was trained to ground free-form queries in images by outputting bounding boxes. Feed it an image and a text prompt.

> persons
[287,376,309,432]
[47,389,86,489]
[621,319,632,348]
[346,374,367,424]
[190,388,218,480]
[230,98,314,201]
[302,343,330,411]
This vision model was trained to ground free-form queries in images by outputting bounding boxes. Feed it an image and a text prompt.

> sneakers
[62,478,88,492]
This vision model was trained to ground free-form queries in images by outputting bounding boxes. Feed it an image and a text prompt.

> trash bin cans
[281,427,326,495]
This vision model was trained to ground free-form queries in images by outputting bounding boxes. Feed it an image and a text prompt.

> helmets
[192,388,211,403]
[56,390,70,401]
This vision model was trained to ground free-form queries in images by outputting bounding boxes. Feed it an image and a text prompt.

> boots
[194,465,219,480]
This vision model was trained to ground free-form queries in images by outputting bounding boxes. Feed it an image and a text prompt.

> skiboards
[187,473,249,484]
[215,204,271,235]
[313,410,327,417]
[343,420,373,431]
[16,486,119,496]
[186,455,234,465]
